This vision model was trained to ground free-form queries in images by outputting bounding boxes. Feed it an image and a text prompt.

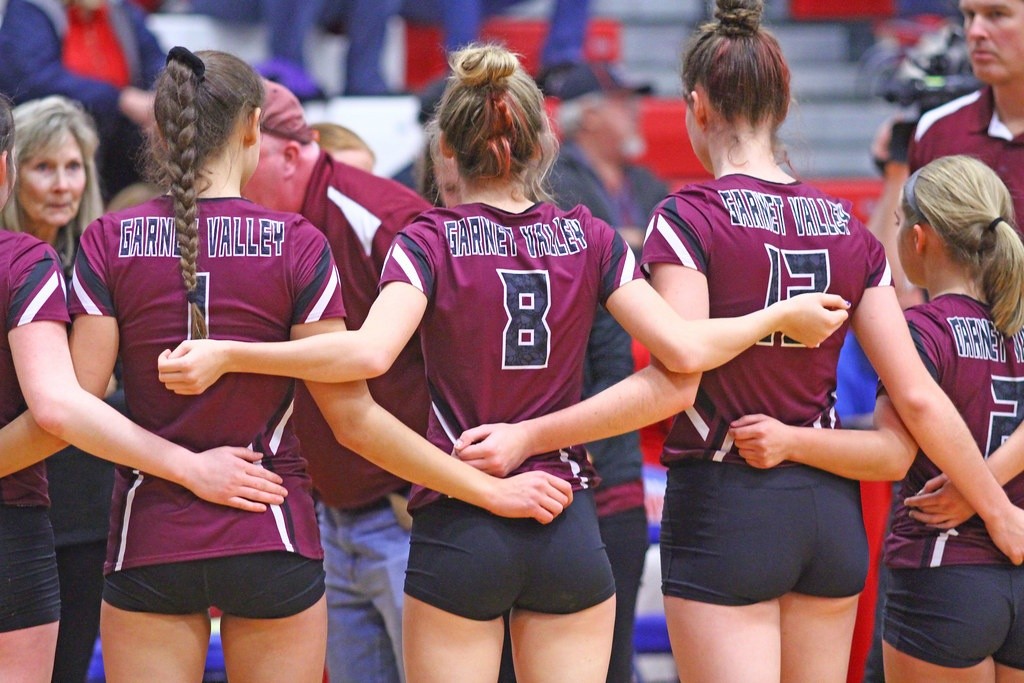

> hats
[553,61,657,98]
[258,80,313,143]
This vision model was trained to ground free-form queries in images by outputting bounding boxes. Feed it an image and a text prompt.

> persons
[158,46,849,683]
[0,0,1024,683]
